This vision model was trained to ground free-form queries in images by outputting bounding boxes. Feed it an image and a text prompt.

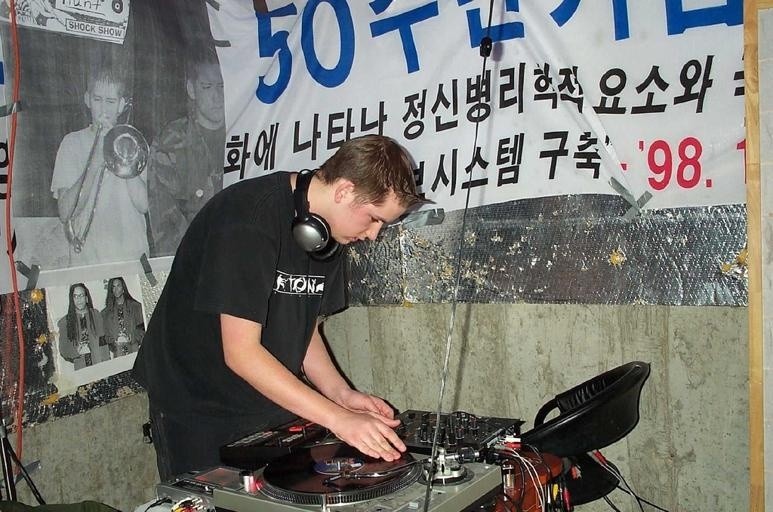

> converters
[290,169,340,263]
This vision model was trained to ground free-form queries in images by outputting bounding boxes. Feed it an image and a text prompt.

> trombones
[62,99,150,253]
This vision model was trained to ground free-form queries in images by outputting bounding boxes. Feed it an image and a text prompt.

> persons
[54,278,111,377]
[145,44,226,261]
[97,273,147,360]
[47,61,153,266]
[125,130,434,492]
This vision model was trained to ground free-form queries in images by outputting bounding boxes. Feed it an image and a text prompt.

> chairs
[516,361,650,512]
